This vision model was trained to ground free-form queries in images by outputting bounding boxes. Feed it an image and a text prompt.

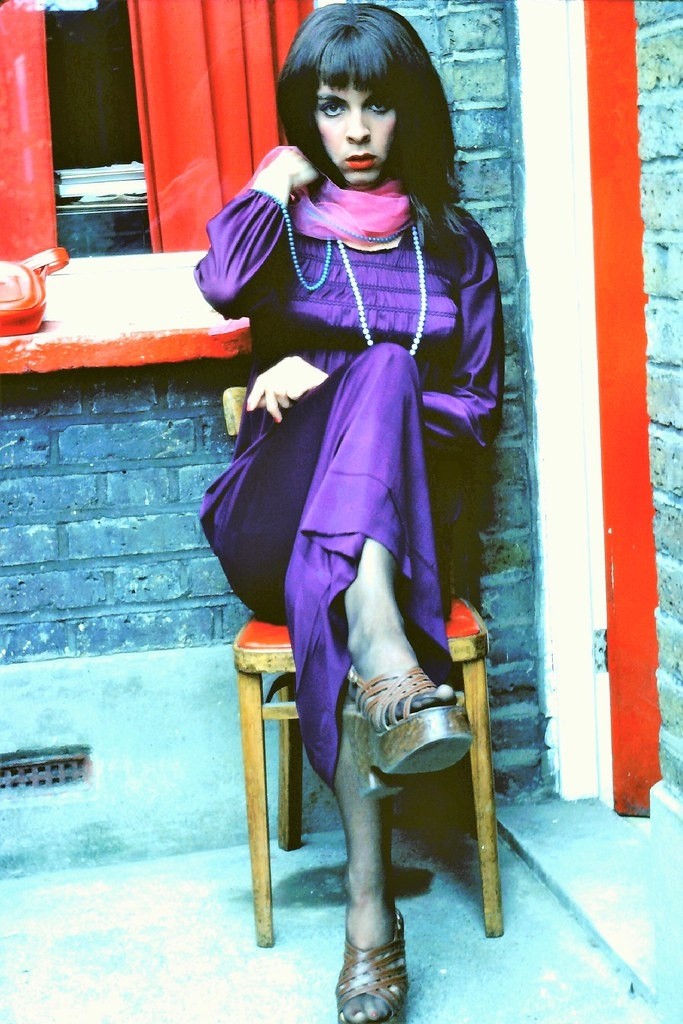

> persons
[200,2,502,1024]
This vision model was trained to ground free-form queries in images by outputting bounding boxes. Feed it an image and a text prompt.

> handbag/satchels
[0,247,69,335]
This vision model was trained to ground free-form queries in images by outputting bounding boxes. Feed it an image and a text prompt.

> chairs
[223,385,504,950]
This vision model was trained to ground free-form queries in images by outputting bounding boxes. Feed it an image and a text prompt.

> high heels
[335,906,407,1024]
[348,664,473,800]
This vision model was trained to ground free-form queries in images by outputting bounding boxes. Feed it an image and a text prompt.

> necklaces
[255,188,427,356]
